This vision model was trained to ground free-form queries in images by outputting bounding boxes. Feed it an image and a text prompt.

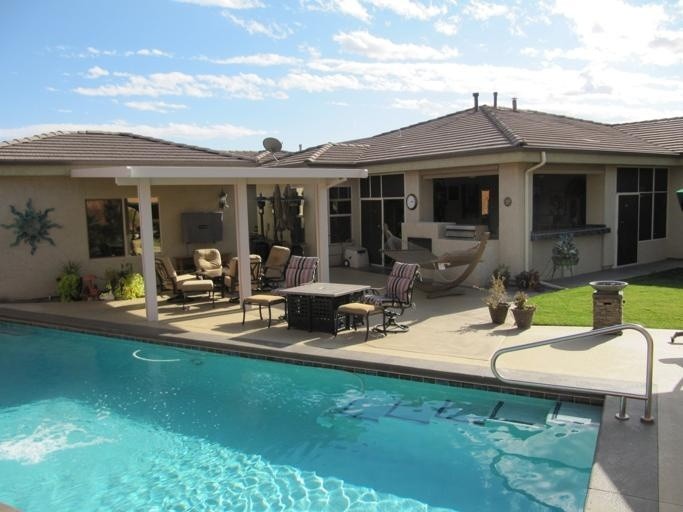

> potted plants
[510,290,536,330]
[473,272,511,325]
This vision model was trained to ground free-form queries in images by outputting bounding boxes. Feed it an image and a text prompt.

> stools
[182,279,215,309]
[334,302,387,342]
[242,295,287,327]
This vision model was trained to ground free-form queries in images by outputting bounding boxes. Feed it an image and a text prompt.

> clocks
[406,194,416,210]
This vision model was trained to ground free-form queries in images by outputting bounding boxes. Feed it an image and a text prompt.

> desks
[270,282,371,337]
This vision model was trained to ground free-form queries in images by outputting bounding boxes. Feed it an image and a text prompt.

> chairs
[155,256,197,302]
[360,261,422,333]
[261,245,291,291]
[223,255,263,302]
[270,255,319,319]
[193,248,223,296]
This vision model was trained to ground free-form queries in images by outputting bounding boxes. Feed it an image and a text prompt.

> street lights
[255,192,268,236]
